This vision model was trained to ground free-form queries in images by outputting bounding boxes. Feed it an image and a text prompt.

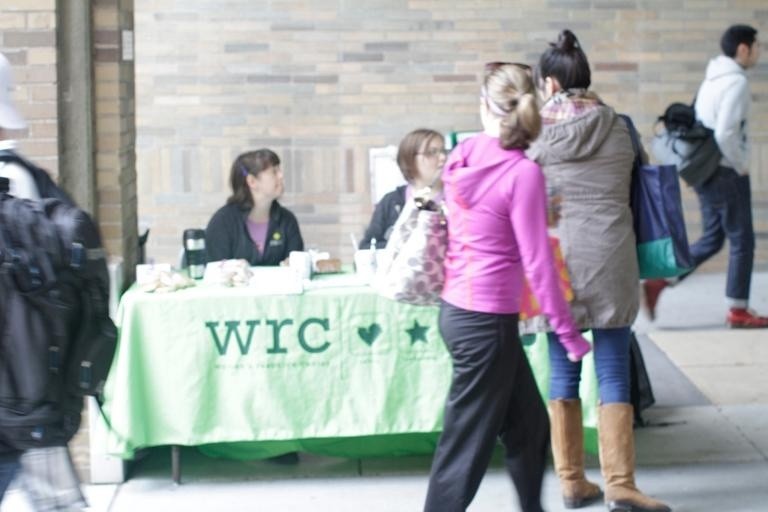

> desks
[102,254,599,482]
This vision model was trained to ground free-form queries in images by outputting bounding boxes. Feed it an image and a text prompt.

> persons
[357,129,448,249]
[0,57,89,512]
[641,25,768,329]
[524,30,674,512]
[422,64,591,512]
[205,149,303,464]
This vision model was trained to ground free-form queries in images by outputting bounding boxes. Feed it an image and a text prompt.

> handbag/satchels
[372,165,449,306]
[652,103,724,186]
[628,163,693,279]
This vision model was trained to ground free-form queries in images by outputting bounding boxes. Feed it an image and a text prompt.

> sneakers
[643,278,667,320]
[726,308,767,329]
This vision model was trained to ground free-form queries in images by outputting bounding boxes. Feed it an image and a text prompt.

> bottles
[182,227,206,281]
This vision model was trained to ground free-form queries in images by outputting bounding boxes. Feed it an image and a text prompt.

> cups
[354,248,386,277]
[135,262,159,293]
[288,251,312,281]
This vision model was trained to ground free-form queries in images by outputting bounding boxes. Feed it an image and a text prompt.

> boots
[546,399,604,508]
[600,403,672,511]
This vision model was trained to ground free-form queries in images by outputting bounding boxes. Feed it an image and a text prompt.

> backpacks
[1,147,117,447]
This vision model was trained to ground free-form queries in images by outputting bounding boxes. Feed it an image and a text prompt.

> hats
[0,52,30,129]
